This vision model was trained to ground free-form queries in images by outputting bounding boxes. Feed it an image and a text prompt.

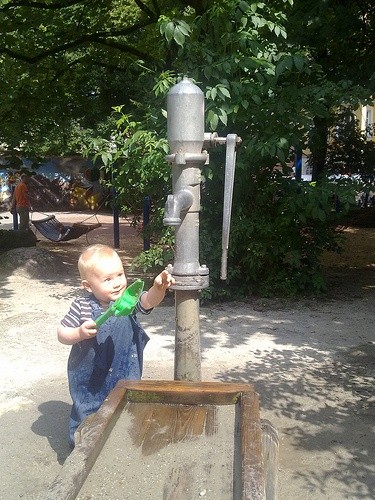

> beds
[26,204,100,242]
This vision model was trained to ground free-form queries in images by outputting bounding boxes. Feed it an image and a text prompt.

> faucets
[162,189,194,227]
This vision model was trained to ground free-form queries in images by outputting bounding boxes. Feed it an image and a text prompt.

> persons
[57,243,176,450]
[9,175,34,233]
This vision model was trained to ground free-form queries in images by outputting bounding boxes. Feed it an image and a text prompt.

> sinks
[41,380,267,500]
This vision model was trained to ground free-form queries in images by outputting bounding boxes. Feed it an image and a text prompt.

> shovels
[93,277,145,327]
